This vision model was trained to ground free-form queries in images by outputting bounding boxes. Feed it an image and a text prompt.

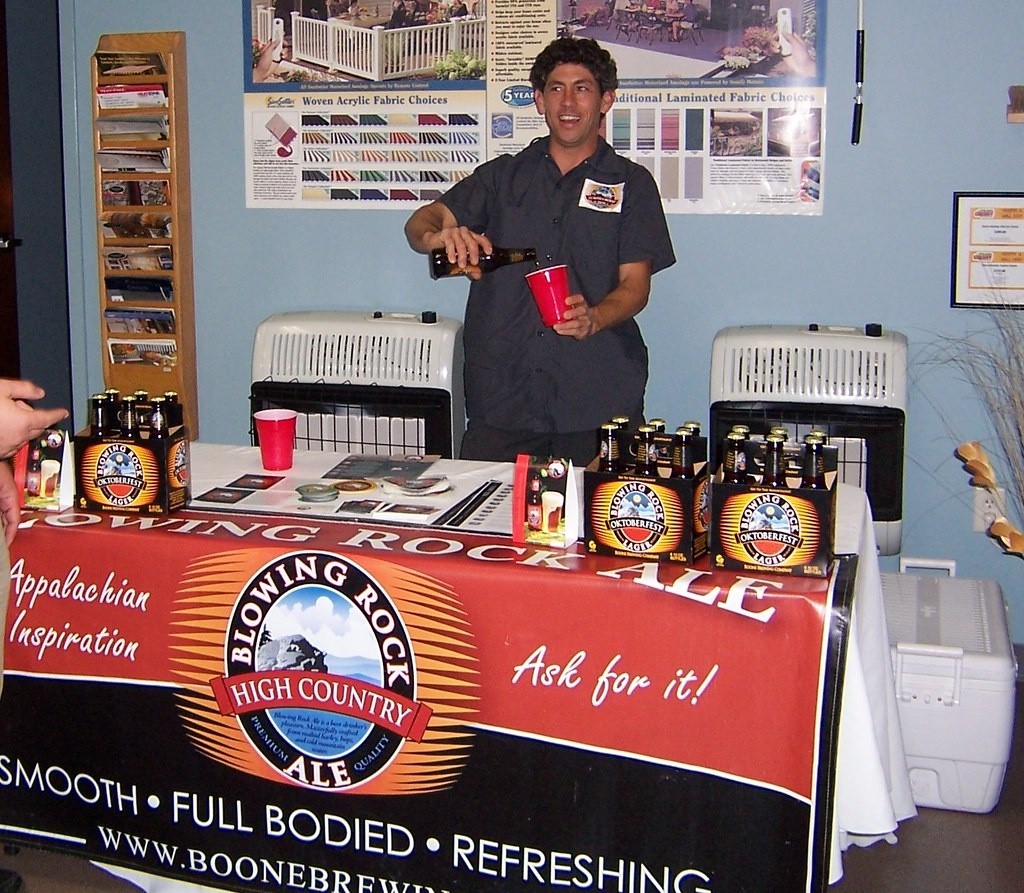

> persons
[402,38,677,467]
[1,376,70,698]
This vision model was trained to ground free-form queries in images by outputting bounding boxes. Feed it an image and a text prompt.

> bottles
[598,423,622,473]
[27,445,42,496]
[648,418,666,433]
[163,390,177,410]
[149,397,169,439]
[723,432,750,484]
[90,394,110,438]
[671,431,695,480]
[634,424,659,477]
[682,421,701,437]
[769,427,788,442]
[731,425,750,439]
[428,246,539,281]
[104,387,120,430]
[761,434,789,487]
[798,437,828,490]
[133,389,151,425]
[528,475,543,531]
[610,415,630,429]
[540,491,564,533]
[119,396,141,439]
[809,431,827,445]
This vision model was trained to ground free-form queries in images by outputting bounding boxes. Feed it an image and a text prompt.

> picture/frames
[949,191,1024,310]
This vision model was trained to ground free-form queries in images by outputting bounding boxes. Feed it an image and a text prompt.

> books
[95,48,178,372]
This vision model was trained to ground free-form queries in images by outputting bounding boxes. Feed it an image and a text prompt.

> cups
[40,459,60,499]
[252,408,298,470]
[525,264,576,327]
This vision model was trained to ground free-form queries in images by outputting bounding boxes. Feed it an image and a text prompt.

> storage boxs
[583,440,711,567]
[877,556,1015,814]
[71,423,191,515]
[707,446,837,578]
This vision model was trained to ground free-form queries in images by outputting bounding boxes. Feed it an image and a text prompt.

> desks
[0,437,861,893]
